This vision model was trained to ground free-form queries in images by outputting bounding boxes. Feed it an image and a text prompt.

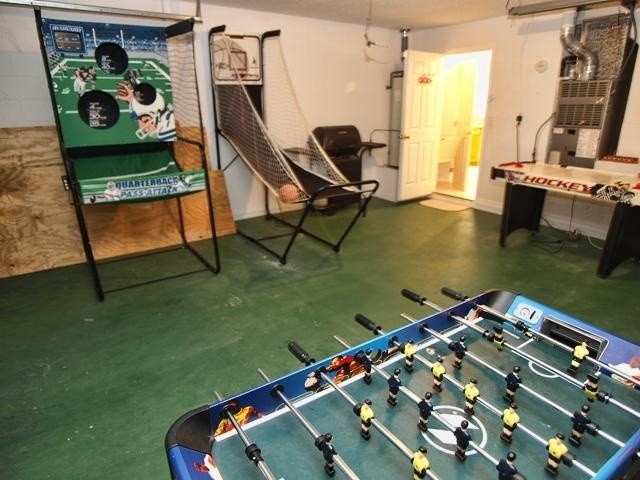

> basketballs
[279,184,299,203]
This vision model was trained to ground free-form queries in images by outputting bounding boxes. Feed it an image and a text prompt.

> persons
[73,63,177,142]
[103,182,121,200]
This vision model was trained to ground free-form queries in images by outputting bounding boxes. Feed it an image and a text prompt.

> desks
[491,160,640,279]
